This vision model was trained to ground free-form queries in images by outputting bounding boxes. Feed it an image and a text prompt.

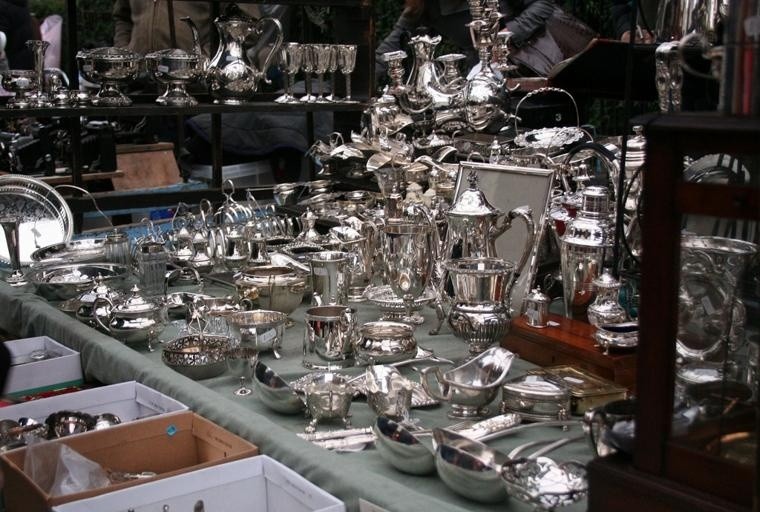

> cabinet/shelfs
[1,93,373,234]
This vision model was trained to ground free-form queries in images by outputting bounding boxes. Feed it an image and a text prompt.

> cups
[23,40,49,93]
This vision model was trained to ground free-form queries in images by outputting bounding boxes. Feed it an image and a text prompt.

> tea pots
[0,93,760,422]
[179,16,284,105]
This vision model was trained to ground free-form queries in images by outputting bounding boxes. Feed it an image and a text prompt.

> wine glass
[74,48,141,108]
[146,48,204,107]
[269,42,358,104]
[2,71,38,109]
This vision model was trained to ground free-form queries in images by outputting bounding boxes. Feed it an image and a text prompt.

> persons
[2,1,655,182]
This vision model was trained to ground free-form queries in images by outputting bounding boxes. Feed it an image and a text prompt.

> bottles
[382,32,471,91]
